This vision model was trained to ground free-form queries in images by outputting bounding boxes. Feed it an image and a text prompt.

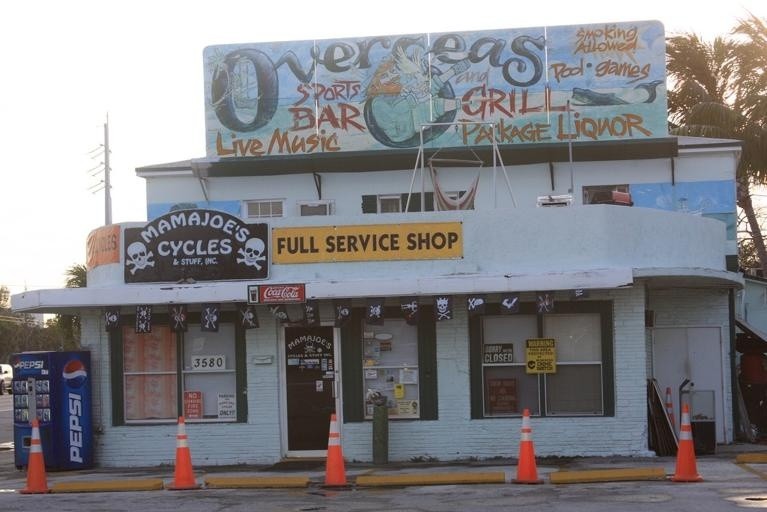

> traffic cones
[321,414,349,488]
[665,387,704,482]
[166,416,202,490]
[19,419,50,494]
[512,408,544,485]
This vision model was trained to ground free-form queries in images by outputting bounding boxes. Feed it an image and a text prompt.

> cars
[0,363,13,396]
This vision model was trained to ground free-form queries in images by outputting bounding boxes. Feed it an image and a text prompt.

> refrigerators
[14,351,92,472]
